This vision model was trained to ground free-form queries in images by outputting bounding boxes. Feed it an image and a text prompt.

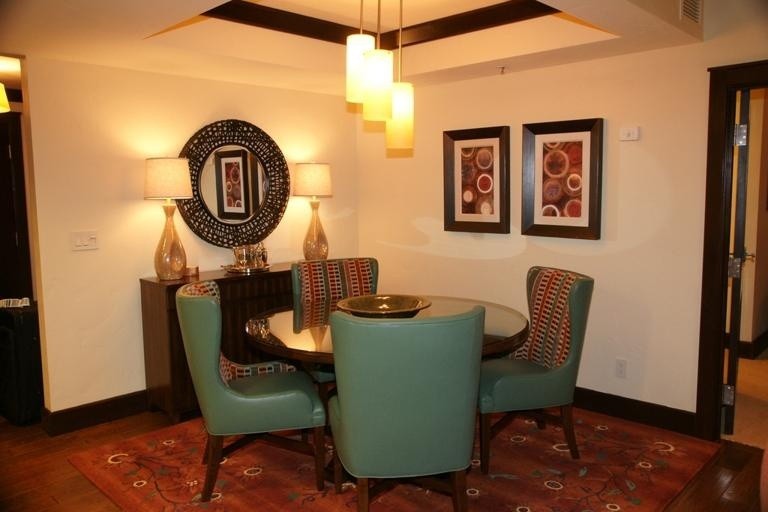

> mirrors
[175,116,290,247]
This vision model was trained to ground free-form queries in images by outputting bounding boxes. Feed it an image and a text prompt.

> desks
[245,292,529,481]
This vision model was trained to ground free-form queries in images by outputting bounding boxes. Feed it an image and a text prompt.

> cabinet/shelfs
[137,261,292,421]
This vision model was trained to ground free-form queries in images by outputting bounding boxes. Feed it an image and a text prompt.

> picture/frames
[249,152,266,213]
[520,116,606,240]
[213,148,250,219]
[442,124,511,233]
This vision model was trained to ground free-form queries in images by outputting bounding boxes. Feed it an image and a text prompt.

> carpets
[71,399,724,512]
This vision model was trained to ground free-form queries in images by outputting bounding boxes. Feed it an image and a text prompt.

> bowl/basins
[334,292,432,320]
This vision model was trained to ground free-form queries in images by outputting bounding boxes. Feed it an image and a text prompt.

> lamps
[291,161,334,260]
[141,157,195,281]
[343,1,415,152]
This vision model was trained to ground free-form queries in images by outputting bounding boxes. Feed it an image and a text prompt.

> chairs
[292,301,341,332]
[173,278,328,501]
[290,258,379,302]
[327,305,485,511]
[471,265,594,473]
